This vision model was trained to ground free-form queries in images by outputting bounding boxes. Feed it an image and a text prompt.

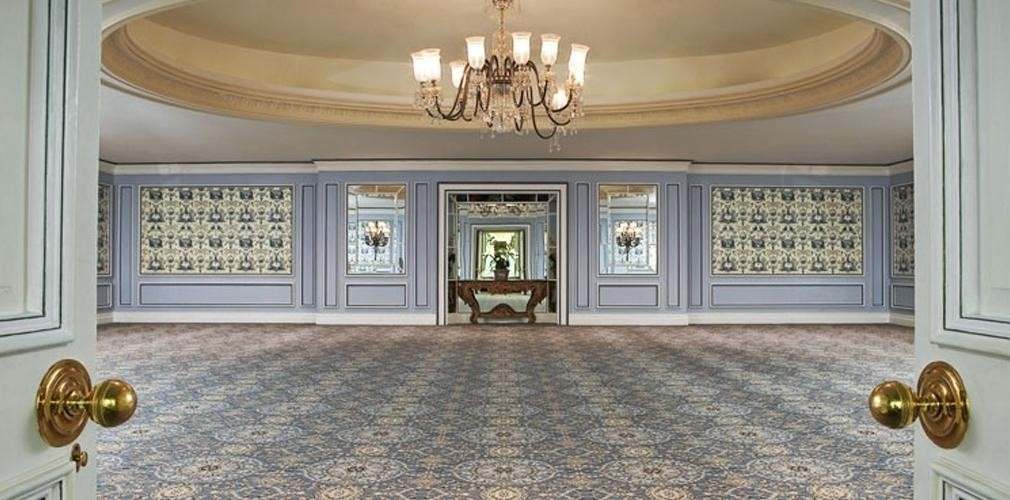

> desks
[457,280,547,324]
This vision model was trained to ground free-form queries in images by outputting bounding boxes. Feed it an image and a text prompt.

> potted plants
[489,254,509,281]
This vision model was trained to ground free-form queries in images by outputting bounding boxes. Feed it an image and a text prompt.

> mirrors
[595,182,660,277]
[344,182,408,278]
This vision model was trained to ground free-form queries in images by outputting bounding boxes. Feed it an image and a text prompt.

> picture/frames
[471,223,531,281]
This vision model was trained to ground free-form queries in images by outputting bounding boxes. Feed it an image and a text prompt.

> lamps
[409,0,591,139]
[614,221,642,262]
[363,220,390,261]
